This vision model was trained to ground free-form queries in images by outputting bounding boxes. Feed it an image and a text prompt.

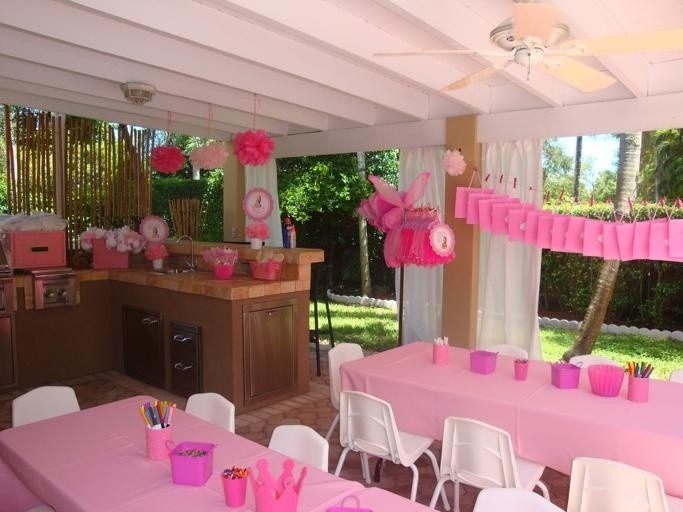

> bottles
[283,216,297,249]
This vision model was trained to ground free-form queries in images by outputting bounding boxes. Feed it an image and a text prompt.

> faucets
[175,234,194,269]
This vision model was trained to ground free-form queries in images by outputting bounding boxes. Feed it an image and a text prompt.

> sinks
[145,267,184,276]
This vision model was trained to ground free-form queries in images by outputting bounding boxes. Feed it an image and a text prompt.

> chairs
[565,456,683,512]
[473,487,566,512]
[429,417,550,512]
[669,369,683,383]
[568,355,622,368]
[486,345,529,359]
[267,424,329,475]
[184,392,236,434]
[332,390,451,512]
[323,342,368,480]
[11,385,80,427]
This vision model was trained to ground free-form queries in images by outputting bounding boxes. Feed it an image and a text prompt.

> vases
[214,264,234,280]
[250,238,263,250]
[152,258,163,269]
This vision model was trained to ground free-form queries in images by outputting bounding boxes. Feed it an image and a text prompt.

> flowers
[189,103,229,170]
[232,93,275,166]
[203,245,239,265]
[246,222,269,240]
[151,110,186,174]
[144,241,169,261]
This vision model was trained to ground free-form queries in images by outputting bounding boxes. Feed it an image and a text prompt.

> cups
[221,470,247,509]
[627,372,651,404]
[432,342,450,365]
[144,424,174,462]
[587,364,625,397]
[513,360,529,381]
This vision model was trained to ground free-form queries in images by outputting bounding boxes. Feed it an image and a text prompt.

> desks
[337,340,683,500]
[0,392,443,512]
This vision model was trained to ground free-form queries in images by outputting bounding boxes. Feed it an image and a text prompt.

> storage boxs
[547,362,583,389]
[92,238,130,270]
[0,232,68,269]
[468,350,496,374]
[163,439,218,487]
[247,260,284,280]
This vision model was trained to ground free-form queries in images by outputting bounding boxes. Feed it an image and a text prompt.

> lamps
[118,81,158,105]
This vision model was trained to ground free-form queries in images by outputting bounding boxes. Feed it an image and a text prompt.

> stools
[312,263,336,376]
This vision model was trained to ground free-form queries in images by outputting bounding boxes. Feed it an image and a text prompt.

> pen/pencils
[434,335,449,346]
[224,464,248,480]
[179,448,206,458]
[515,359,527,363]
[628,360,655,378]
[139,399,176,430]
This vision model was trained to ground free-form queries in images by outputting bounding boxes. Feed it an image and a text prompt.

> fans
[372,0,683,96]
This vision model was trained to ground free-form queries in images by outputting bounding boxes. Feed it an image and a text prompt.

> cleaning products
[284,216,297,248]
